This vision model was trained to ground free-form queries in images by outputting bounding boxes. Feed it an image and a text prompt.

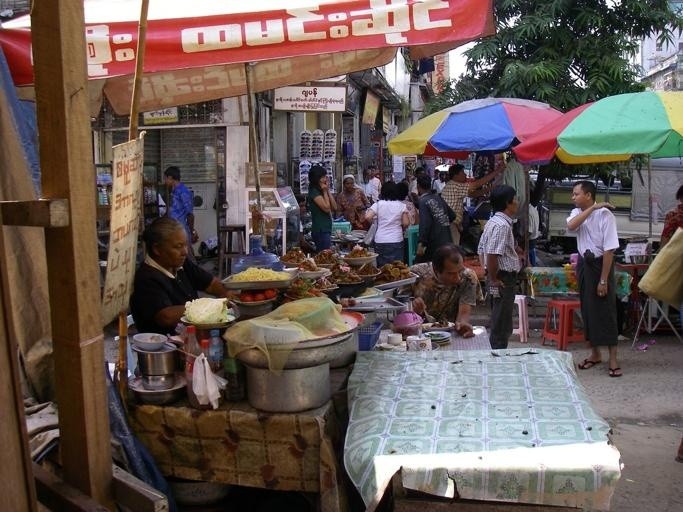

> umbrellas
[386,94,566,172]
[510,90,682,239]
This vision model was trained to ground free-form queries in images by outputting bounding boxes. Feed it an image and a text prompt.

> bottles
[222,356,247,402]
[231,233,283,274]
[183,325,225,370]
[96,169,112,205]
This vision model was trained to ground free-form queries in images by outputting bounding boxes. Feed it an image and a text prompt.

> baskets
[357,320,385,352]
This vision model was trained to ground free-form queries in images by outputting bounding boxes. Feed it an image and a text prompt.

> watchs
[599,278,607,285]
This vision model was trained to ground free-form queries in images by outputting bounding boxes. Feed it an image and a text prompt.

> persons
[477,184,523,349]
[130,216,240,335]
[565,180,624,377]
[308,163,501,267]
[656,184,683,254]
[163,164,194,260]
[408,247,483,339]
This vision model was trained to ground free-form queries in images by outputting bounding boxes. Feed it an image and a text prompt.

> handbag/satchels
[362,202,380,247]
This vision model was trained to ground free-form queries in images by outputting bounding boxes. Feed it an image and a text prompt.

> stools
[541,300,586,351]
[408,225,419,266]
[510,294,529,344]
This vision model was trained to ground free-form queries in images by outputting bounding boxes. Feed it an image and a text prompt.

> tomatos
[240,289,277,302]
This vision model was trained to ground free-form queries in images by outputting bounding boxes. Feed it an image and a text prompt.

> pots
[243,363,329,412]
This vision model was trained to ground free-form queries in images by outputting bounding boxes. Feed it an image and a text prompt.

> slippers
[608,366,623,378]
[578,357,603,370]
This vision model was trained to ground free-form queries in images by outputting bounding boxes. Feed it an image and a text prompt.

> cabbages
[183,297,229,323]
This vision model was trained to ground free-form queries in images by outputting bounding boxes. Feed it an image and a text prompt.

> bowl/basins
[628,254,649,265]
[235,334,354,369]
[650,253,657,264]
[126,377,187,405]
[132,333,167,350]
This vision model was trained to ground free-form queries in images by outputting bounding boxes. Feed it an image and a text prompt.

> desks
[342,349,625,511]
[524,266,633,332]
[374,325,492,350]
[123,361,356,512]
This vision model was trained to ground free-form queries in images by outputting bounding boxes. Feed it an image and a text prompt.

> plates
[281,252,382,291]
[178,311,241,326]
[231,289,279,307]
[379,320,455,352]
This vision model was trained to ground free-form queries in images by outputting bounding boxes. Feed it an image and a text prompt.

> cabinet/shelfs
[245,162,300,269]
